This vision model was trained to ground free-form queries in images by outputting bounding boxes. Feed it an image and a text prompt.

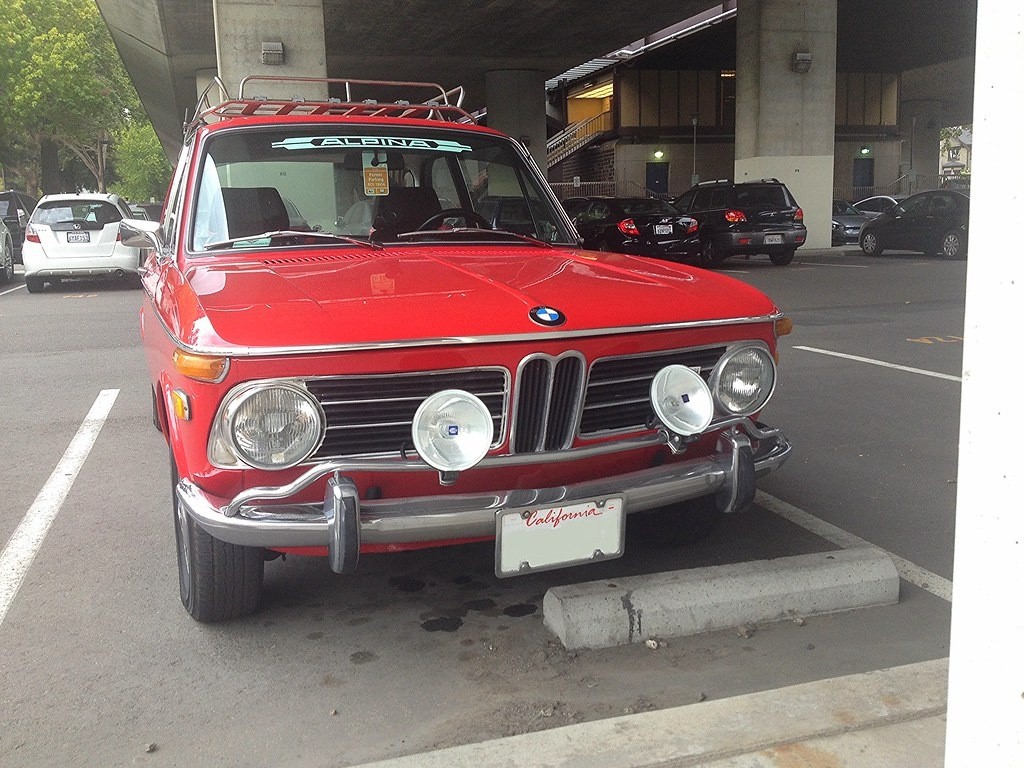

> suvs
[135,71,795,630]
[671,178,809,269]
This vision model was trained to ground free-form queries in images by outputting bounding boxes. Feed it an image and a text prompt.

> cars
[197,191,467,242]
[21,192,143,293]
[845,195,924,220]
[832,198,870,246]
[473,193,702,264]
[859,187,969,260]
[0,188,167,284]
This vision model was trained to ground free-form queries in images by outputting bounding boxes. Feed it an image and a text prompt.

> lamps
[261,42,285,65]
[654,150,664,158]
[860,146,870,155]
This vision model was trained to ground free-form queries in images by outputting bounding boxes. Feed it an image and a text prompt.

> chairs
[207,187,291,246]
[930,197,946,213]
[368,186,444,244]
